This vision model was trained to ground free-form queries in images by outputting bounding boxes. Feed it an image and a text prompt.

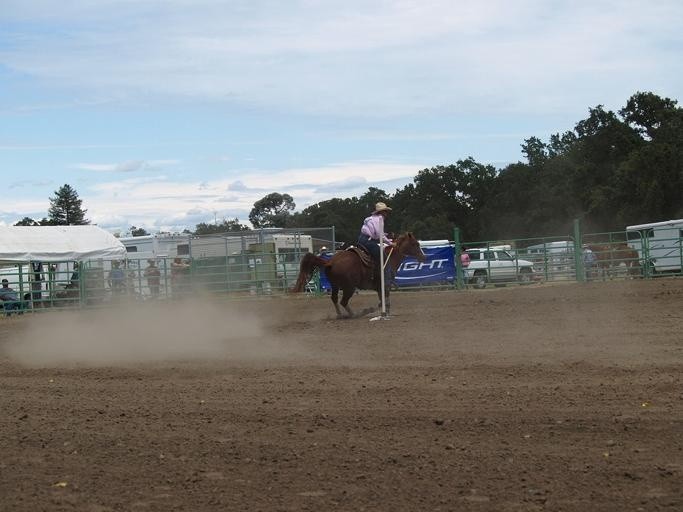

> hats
[371,202,392,214]
[319,246,329,252]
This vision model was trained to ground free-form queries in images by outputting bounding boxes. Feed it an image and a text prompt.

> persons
[170,255,193,298]
[144,259,161,298]
[359,202,396,279]
[48,264,58,280]
[28,262,45,307]
[108,259,124,301]
[460,247,471,289]
[582,243,596,280]
[122,258,136,297]
[0,279,21,315]
[318,245,332,296]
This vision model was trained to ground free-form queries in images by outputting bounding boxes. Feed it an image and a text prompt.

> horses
[582,244,640,282]
[293,231,425,322]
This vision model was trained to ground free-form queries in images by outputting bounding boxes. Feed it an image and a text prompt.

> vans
[625,218,682,278]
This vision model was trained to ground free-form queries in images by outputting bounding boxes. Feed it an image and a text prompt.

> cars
[460,248,535,290]
